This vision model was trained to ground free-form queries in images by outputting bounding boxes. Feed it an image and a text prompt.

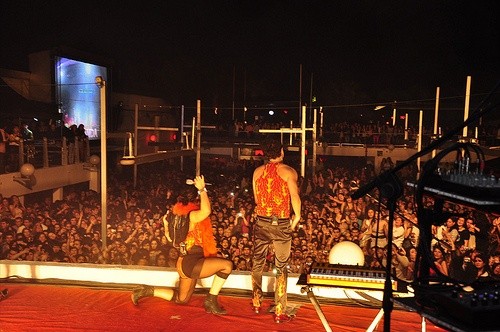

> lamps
[83,155,100,170]
[13,164,35,184]
[329,241,364,266]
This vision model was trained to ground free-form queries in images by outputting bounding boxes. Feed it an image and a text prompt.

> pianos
[296,261,398,332]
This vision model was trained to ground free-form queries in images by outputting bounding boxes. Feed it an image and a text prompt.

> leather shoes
[274,315,294,324]
[251,305,260,313]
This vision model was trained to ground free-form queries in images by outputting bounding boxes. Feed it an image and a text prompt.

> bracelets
[197,188,207,194]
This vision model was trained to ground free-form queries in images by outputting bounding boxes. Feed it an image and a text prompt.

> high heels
[129,285,153,306]
[204,293,228,316]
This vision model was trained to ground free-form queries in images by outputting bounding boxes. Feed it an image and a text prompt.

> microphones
[185,179,212,185]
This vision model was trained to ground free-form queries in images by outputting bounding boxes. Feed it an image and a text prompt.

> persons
[0,119,87,173]
[252,140,302,321]
[238,120,455,145]
[0,158,500,281]
[132,175,233,315]
